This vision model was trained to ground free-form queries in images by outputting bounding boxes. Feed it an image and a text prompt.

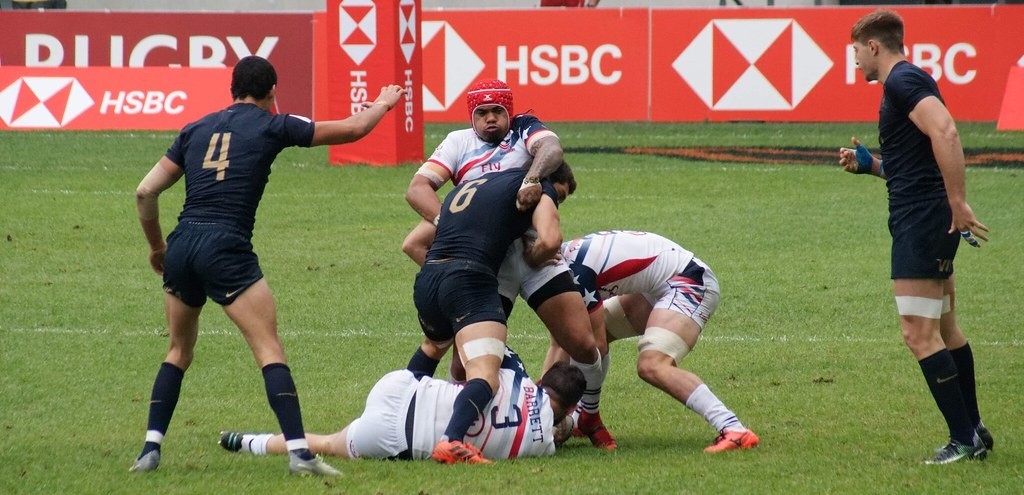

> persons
[221,361,587,461]
[838,10,994,465]
[401,77,617,461]
[539,228,757,454]
[129,55,410,480]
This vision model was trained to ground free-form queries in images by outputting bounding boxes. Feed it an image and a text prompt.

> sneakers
[578,411,619,450]
[132,452,159,471]
[928,423,994,464]
[218,428,253,454]
[703,429,759,452]
[288,452,343,480]
[431,439,492,465]
